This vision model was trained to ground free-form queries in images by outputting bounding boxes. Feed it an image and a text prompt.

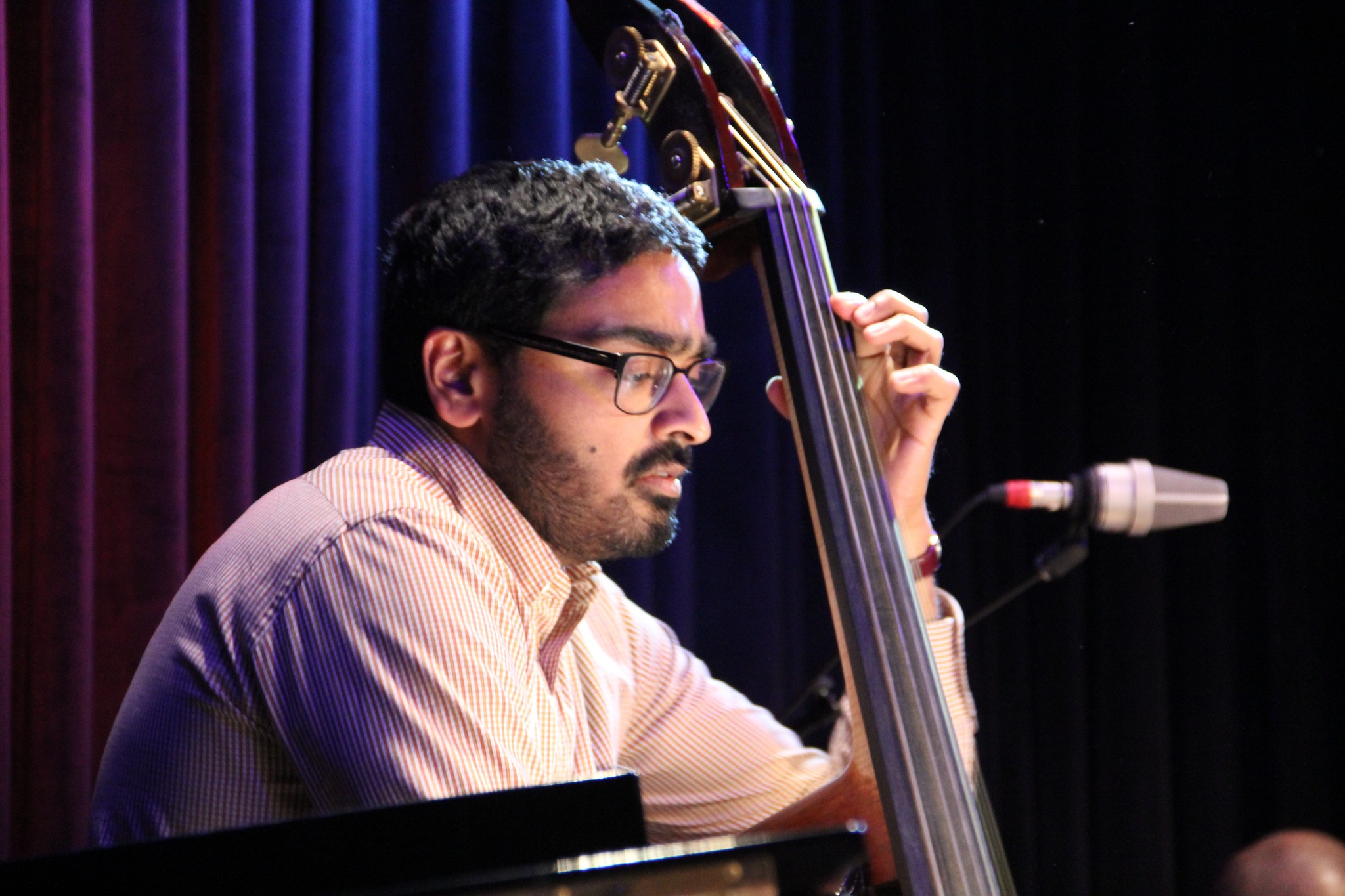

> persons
[86,154,977,885]
[1215,829,1345,896]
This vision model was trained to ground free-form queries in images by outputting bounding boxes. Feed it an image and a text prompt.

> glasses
[464,325,727,415]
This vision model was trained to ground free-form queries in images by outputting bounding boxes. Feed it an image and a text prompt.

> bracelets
[908,530,942,581]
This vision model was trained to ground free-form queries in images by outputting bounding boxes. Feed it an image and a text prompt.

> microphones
[992,457,1229,536]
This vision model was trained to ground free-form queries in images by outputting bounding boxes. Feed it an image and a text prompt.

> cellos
[574,0,1019,896]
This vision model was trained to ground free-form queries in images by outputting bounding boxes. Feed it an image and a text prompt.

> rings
[891,352,903,363]
